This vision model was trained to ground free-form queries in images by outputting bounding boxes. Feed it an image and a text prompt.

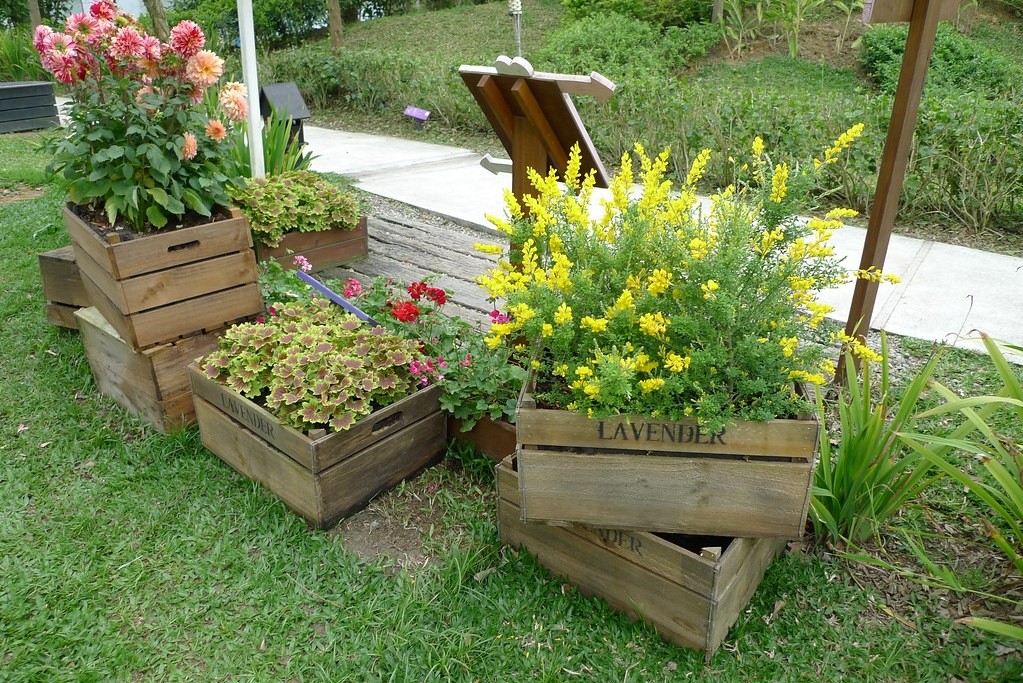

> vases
[62,199,264,352]
[516,337,821,541]
[448,412,516,463]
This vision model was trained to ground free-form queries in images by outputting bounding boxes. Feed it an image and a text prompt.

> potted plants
[186,299,448,532]
[225,170,368,274]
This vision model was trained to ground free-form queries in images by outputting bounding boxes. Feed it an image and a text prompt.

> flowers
[255,248,527,432]
[33,0,248,233]
[474,122,901,436]
